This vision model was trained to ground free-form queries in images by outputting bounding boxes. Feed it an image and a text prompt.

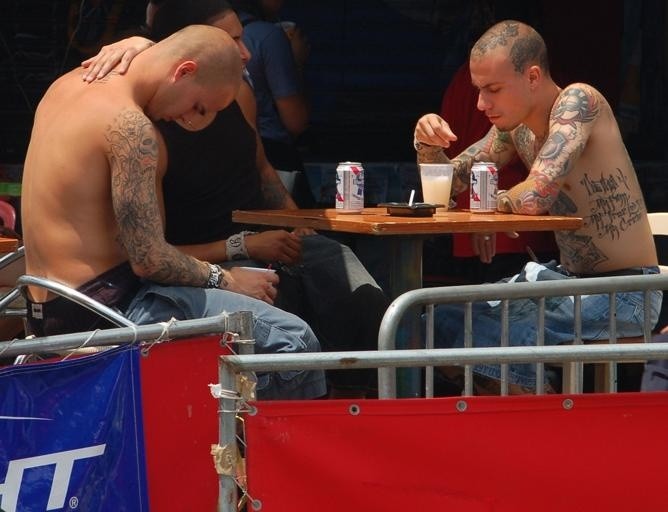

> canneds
[335,161,365,214]
[470,161,498,213]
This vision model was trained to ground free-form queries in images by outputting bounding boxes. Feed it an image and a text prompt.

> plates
[377,203,446,217]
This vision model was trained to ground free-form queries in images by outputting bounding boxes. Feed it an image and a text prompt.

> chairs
[560,212,668,392]
[4,272,145,353]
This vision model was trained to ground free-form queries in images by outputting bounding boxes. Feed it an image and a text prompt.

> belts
[592,267,644,277]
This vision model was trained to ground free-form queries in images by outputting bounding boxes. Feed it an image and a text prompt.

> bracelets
[204,263,221,286]
[224,228,254,265]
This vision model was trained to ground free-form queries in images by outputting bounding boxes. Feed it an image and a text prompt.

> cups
[419,163,454,211]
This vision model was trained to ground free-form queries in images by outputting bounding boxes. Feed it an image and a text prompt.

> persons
[411,19,664,394]
[19,24,328,401]
[141,1,397,386]
[232,1,319,209]
[438,0,554,281]
[65,0,143,68]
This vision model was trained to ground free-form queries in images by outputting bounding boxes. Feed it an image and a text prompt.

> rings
[482,236,491,240]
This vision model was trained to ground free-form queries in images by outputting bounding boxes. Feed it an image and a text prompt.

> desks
[233,193,585,396]
